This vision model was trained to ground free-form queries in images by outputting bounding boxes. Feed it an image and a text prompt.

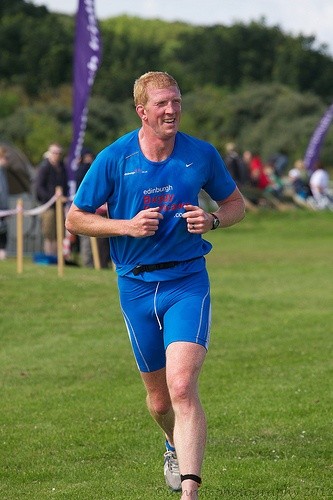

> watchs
[208,212,220,230]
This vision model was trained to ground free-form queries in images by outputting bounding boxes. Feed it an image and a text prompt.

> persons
[0,145,106,266]
[64,71,247,500]
[223,140,331,223]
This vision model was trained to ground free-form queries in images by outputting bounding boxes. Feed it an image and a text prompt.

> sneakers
[162,444,182,491]
